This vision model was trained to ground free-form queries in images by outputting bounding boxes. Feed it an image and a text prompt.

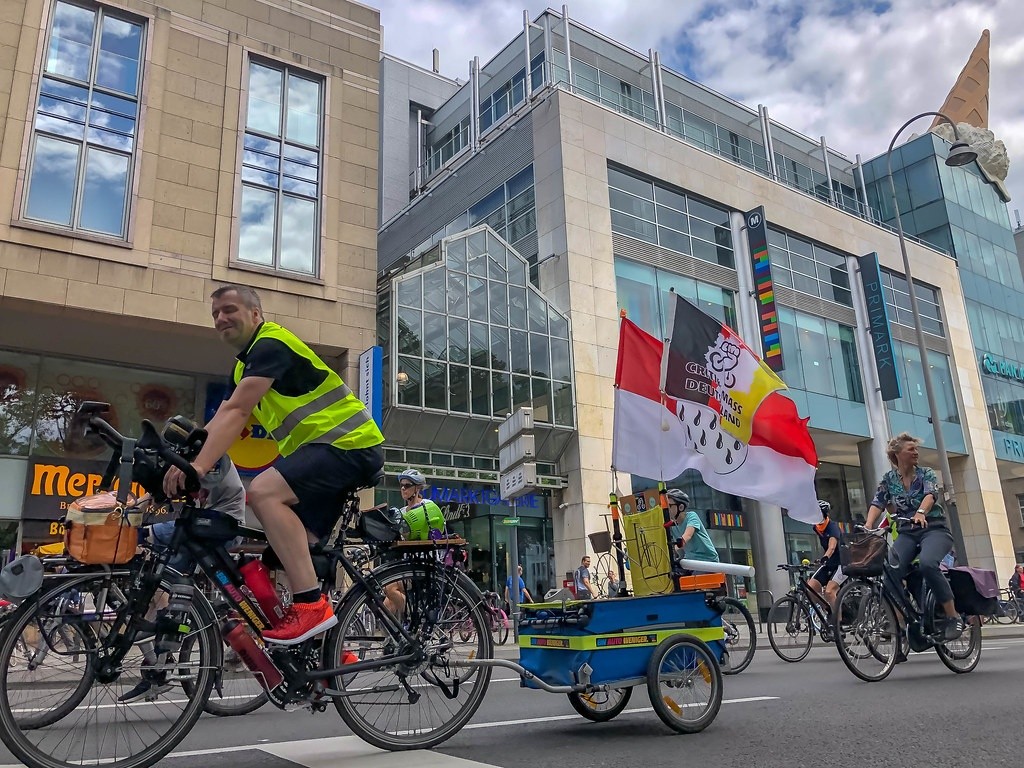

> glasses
[400,482,416,488]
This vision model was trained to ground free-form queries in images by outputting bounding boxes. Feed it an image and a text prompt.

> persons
[1009,564,1024,622]
[575,556,596,600]
[663,488,720,594]
[806,499,848,640]
[377,469,448,636]
[116,283,388,705]
[608,571,619,598]
[505,565,535,620]
[863,432,965,639]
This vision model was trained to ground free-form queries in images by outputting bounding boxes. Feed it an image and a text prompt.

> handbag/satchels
[64,488,144,565]
[949,566,1001,615]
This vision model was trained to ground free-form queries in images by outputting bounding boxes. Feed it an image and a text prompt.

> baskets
[839,532,887,576]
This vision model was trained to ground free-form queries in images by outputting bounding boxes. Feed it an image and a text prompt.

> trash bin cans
[544,587,575,603]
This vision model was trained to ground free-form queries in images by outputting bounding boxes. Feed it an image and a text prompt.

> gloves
[814,556,830,567]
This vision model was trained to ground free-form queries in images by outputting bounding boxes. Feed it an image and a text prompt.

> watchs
[916,507,927,517]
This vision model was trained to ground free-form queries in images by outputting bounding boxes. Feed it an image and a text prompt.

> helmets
[817,500,830,514]
[666,488,690,506]
[398,469,426,485]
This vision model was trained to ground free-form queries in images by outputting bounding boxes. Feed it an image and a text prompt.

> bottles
[159,573,196,650]
[220,615,283,693]
[238,549,283,628]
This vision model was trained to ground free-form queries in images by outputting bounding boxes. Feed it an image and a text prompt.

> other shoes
[404,608,425,630]
[881,625,906,639]
[1018,622,1024,624]
[340,648,363,664]
[840,627,846,638]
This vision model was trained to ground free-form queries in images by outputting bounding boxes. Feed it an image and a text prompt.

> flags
[614,319,822,525]
[660,293,786,477]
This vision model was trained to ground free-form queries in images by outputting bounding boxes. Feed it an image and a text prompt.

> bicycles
[1,402,494,768]
[831,513,982,682]
[28,611,56,670]
[991,600,1018,624]
[767,558,880,663]
[8,638,17,667]
[714,596,757,674]
[458,590,509,646]
[982,586,1024,625]
[588,514,672,599]
[330,600,484,686]
[0,590,277,717]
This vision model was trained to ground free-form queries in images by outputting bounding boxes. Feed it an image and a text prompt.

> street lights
[887,111,978,567]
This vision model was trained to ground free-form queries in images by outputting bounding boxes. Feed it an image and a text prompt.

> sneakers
[117,671,174,703]
[944,612,963,640]
[262,592,339,645]
